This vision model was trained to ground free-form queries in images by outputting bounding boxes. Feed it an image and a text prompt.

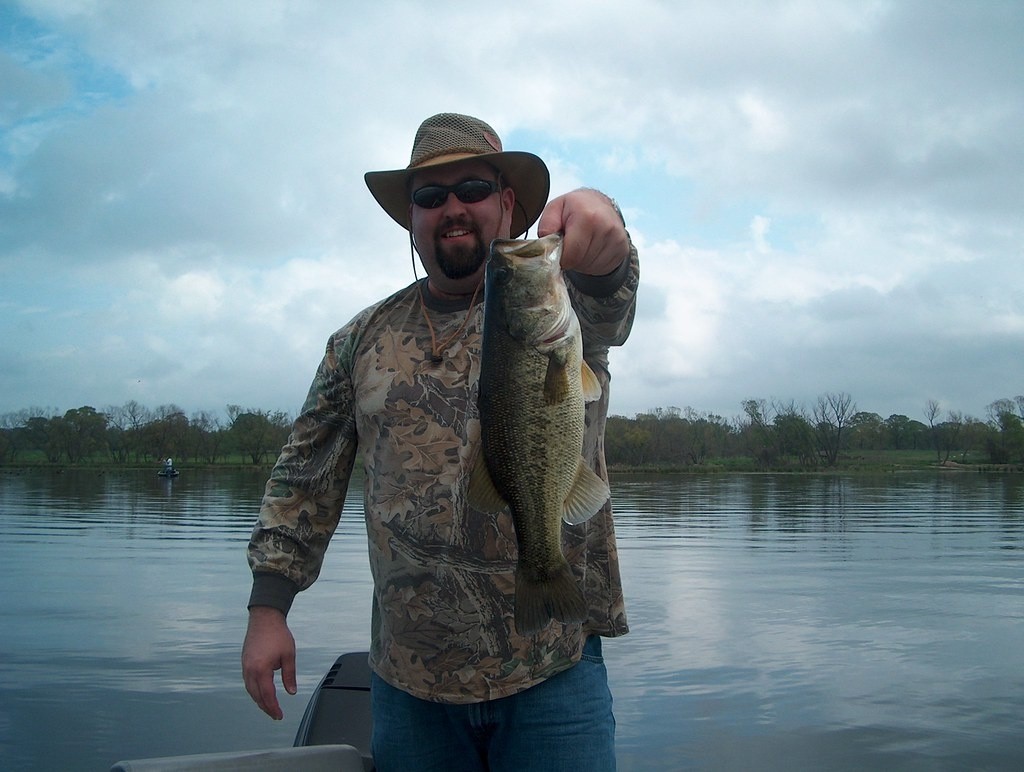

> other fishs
[477,230,609,637]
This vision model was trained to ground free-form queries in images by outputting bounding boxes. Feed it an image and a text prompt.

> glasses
[410,179,500,209]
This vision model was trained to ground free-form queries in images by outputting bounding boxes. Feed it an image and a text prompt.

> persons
[161,456,172,470]
[241,115,640,772]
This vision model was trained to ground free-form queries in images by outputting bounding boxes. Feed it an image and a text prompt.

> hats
[364,113,551,239]
